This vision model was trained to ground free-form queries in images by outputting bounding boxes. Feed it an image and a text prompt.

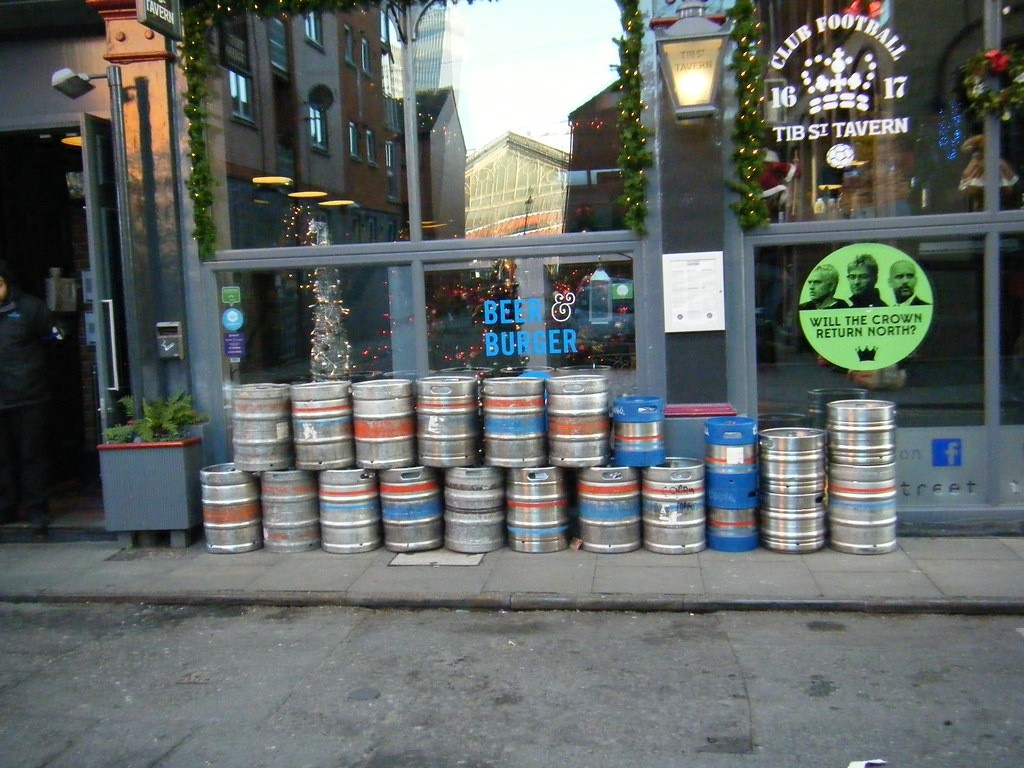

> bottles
[910,122,943,214]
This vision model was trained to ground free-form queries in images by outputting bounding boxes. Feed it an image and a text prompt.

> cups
[842,171,876,218]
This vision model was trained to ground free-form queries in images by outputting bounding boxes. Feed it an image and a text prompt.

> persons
[846,254,889,308]
[887,260,932,307]
[800,264,850,310]
[0,273,58,534]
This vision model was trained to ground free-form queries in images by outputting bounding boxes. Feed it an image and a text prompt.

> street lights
[48,63,147,433]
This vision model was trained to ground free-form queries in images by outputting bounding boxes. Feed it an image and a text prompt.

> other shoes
[0,504,19,525]
[30,506,48,532]
